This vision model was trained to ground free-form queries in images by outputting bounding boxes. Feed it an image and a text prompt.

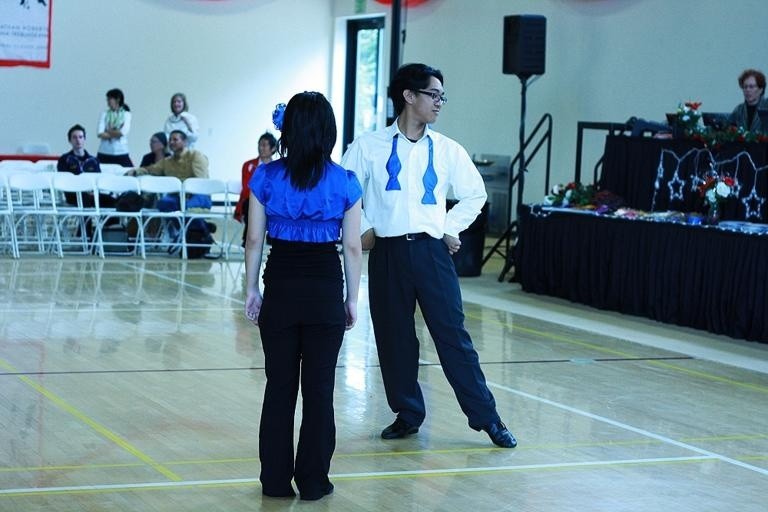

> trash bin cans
[446,198,491,277]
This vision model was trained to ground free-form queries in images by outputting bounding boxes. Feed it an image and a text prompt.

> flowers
[550,174,623,215]
[678,99,766,147]
[690,160,735,206]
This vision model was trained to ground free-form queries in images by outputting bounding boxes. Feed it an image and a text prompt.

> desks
[516,130,766,343]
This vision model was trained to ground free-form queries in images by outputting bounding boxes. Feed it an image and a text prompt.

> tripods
[482,76,529,269]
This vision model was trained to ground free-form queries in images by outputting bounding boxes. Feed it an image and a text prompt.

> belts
[393,234,428,241]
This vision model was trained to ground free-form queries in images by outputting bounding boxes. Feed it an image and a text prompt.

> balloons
[271,102,286,133]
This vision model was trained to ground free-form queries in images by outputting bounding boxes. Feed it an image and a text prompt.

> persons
[96,87,135,168]
[124,129,213,253]
[727,68,768,134]
[338,62,518,449]
[56,123,120,237]
[126,130,172,237]
[234,131,280,248]
[243,90,365,502]
[164,92,199,155]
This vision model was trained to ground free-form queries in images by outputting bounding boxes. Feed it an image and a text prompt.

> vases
[706,200,720,226]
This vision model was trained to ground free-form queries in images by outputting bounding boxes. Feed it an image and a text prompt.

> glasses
[418,90,447,104]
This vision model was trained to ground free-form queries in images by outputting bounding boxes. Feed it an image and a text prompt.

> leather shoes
[382,419,419,438]
[468,419,517,447]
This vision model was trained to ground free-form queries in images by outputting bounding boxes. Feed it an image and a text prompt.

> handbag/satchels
[178,219,214,258]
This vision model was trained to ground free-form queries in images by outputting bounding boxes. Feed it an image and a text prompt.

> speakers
[503,14,547,74]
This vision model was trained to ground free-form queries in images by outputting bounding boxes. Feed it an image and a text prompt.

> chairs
[0,155,246,263]
